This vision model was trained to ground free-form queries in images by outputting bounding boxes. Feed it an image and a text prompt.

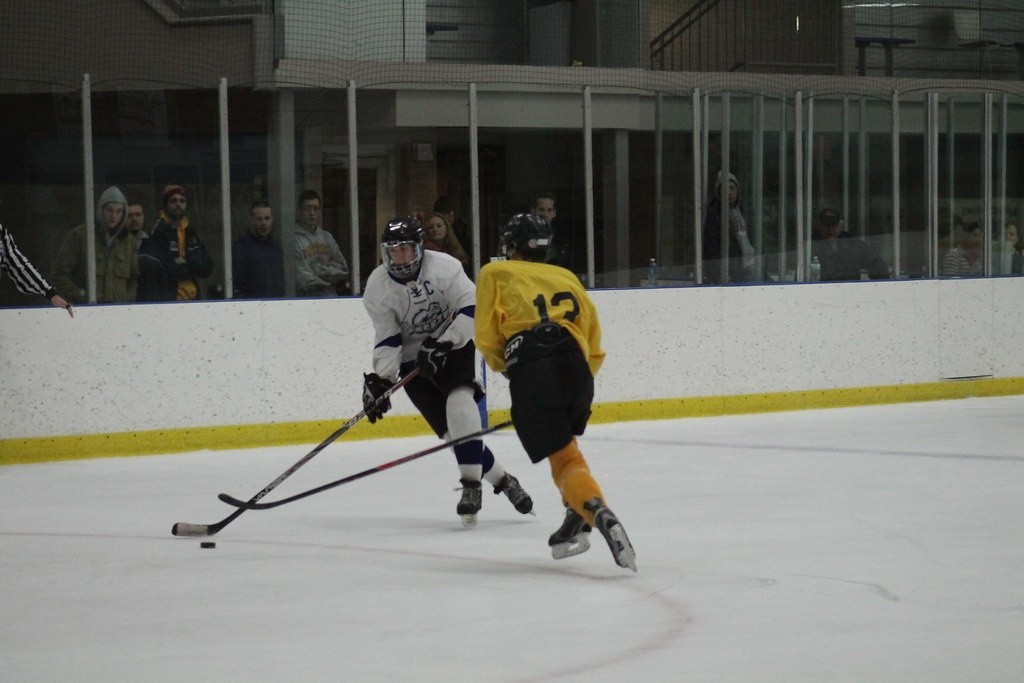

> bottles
[811,257,821,283]
[647,258,658,289]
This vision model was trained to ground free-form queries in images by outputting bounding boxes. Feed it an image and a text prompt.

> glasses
[302,206,320,211]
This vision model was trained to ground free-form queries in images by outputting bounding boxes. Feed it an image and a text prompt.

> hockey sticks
[218,419,516,511]
[171,366,421,537]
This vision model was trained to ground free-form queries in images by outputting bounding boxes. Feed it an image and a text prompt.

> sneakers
[593,507,639,573]
[549,505,592,558]
[492,471,536,518]
[454,477,482,527]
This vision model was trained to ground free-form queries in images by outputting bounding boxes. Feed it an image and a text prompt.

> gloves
[362,371,393,423]
[418,335,454,377]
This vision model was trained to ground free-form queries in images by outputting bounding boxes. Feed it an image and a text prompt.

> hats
[164,183,188,199]
[817,209,844,220]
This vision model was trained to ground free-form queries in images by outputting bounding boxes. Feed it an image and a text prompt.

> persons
[1,187,574,309]
[938,221,1024,277]
[360,216,536,527]
[798,208,891,284]
[475,213,640,571]
[706,169,763,283]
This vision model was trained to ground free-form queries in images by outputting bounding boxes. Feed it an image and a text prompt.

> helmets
[380,215,425,282]
[501,213,552,258]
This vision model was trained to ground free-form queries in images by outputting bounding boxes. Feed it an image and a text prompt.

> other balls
[201,542,216,548]
